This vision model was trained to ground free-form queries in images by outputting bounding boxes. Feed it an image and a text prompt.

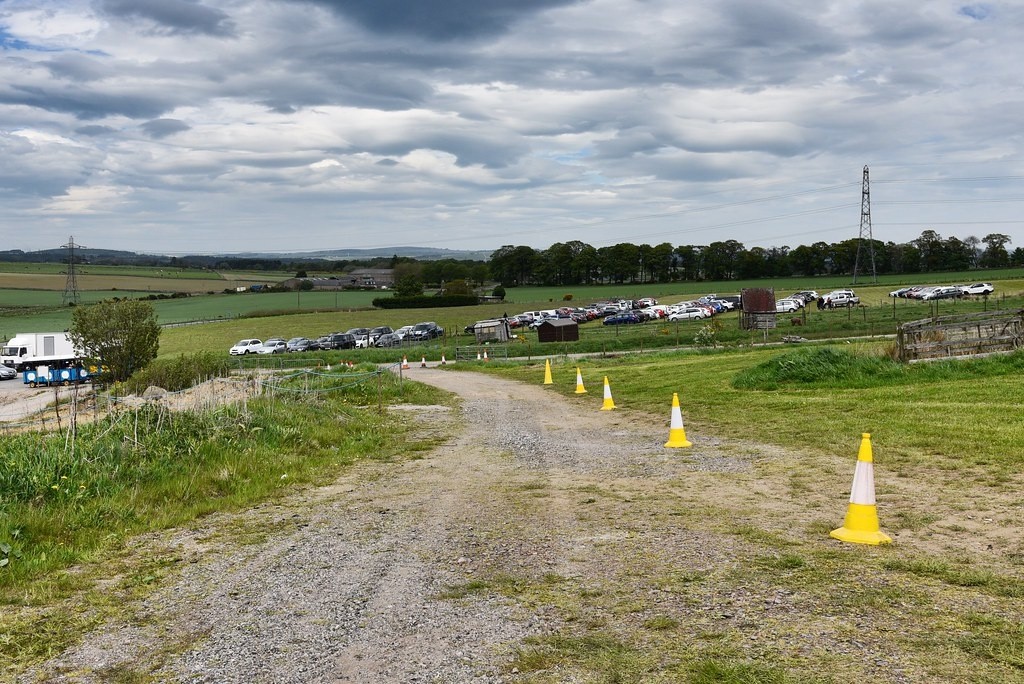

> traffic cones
[600,375,618,412]
[663,392,693,448]
[827,431,893,547]
[400,355,411,370]
[483,348,489,362]
[476,351,481,361]
[441,353,446,365]
[574,366,588,394]
[420,354,428,369]
[543,358,553,384]
[340,359,353,370]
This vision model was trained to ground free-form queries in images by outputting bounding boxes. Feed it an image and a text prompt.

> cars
[257,319,445,355]
[887,282,995,301]
[0,364,18,380]
[463,287,861,334]
[228,338,264,356]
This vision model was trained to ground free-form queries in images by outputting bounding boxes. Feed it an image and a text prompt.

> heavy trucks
[0,331,96,372]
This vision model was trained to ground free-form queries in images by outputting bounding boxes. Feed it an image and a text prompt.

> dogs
[791,318,802,326]
